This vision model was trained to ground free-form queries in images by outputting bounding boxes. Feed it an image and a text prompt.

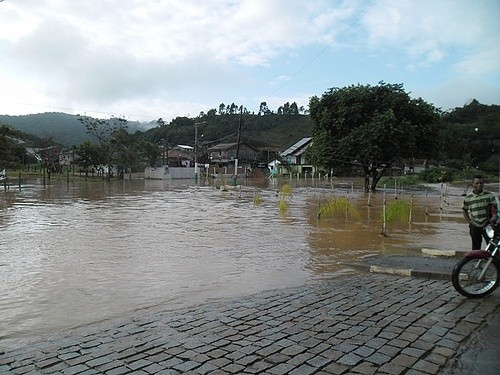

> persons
[461,175,498,251]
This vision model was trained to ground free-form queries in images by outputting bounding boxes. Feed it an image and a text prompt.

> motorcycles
[452,221,500,297]
